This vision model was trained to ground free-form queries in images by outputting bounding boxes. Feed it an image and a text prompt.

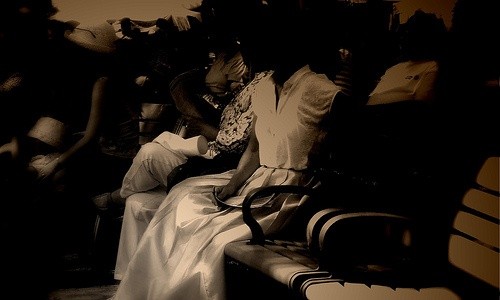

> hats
[65,18,117,53]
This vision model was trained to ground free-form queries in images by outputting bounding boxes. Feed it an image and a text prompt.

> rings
[42,174,45,176]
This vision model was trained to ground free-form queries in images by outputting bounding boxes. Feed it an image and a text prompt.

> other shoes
[91,192,125,211]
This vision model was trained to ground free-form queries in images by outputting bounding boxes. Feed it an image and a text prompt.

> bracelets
[56,157,61,165]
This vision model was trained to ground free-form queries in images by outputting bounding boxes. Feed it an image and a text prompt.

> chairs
[300,154,499,300]
[223,142,421,292]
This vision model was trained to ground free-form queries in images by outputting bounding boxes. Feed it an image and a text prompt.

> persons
[93,30,270,280]
[112,18,345,300]
[36,19,139,181]
